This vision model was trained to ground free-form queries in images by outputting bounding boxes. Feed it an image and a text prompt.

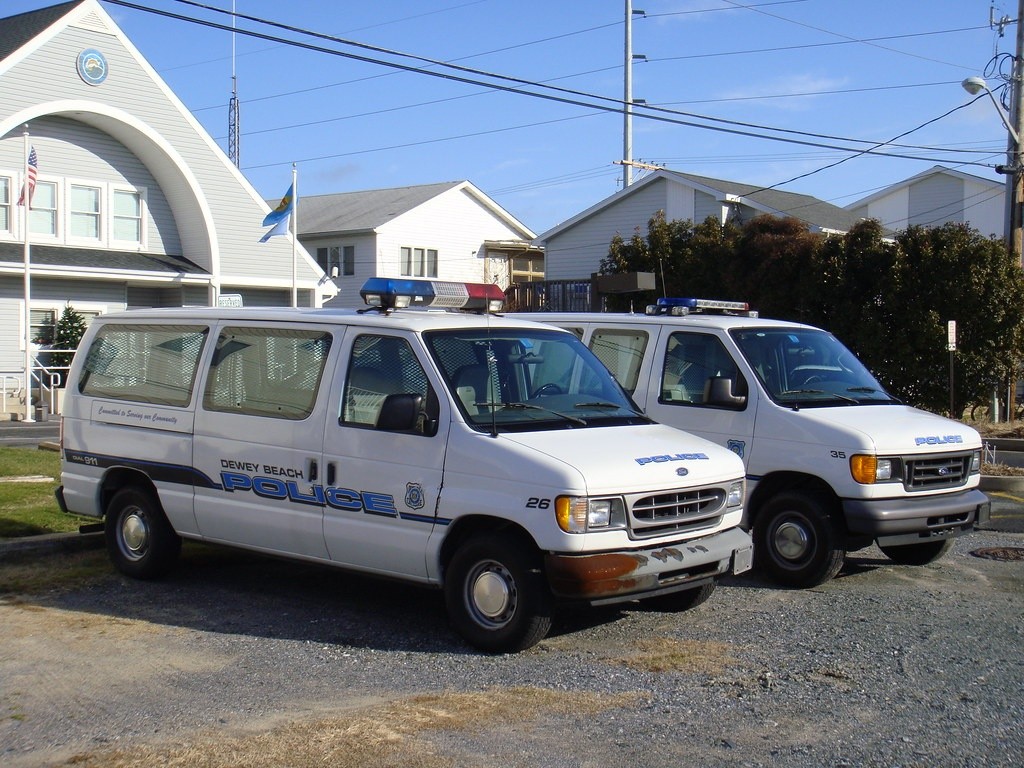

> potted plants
[40,298,89,415]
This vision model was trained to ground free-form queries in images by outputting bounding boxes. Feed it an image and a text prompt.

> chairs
[452,364,499,416]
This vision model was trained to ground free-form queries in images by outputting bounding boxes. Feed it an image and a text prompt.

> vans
[55,276,757,657]
[489,297,992,591]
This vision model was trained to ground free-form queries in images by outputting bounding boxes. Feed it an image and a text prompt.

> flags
[17,146,38,208]
[258,181,298,243]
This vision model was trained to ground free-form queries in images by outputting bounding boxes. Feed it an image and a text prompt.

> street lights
[959,76,1024,425]
